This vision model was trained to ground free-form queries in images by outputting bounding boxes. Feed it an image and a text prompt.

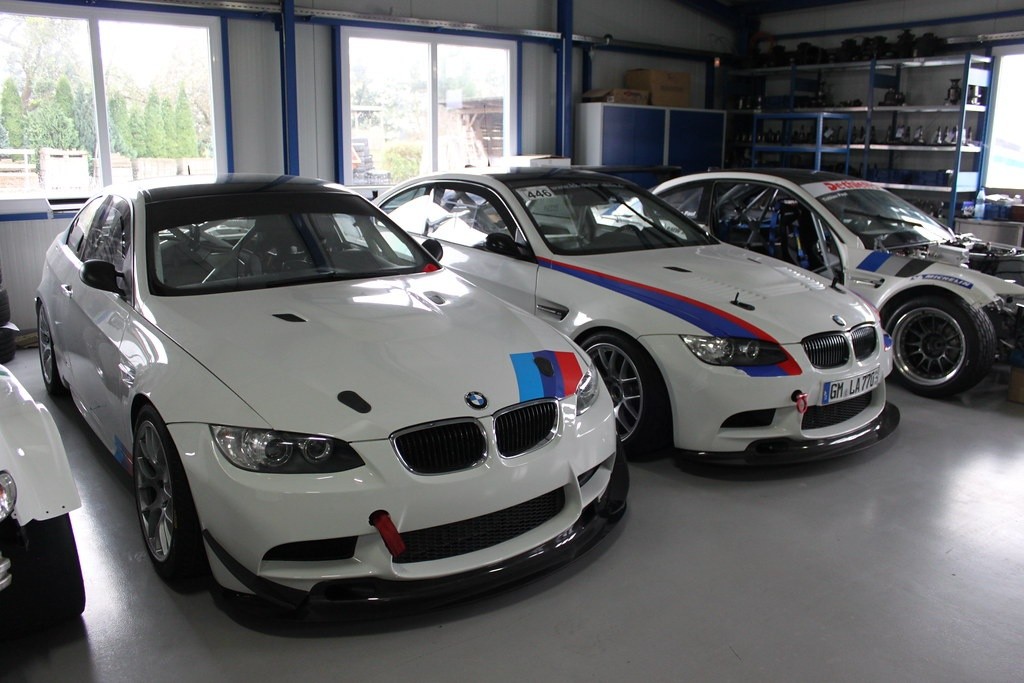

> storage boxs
[603,70,693,107]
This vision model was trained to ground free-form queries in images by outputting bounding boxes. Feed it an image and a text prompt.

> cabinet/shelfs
[721,52,1001,230]
[574,103,727,182]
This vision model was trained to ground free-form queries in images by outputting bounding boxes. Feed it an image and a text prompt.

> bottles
[974,188,986,219]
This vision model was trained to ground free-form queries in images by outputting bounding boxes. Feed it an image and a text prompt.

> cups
[1015,195,1020,203]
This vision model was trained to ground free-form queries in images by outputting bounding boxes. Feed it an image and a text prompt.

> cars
[358,166,902,470]
[35,173,632,622]
[596,165,1024,398]
[0,364,86,632]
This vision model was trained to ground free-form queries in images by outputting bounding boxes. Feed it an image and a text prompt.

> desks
[955,218,1024,248]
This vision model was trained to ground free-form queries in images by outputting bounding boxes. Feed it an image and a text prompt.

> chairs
[238,212,375,277]
[159,230,263,287]
[768,200,812,270]
